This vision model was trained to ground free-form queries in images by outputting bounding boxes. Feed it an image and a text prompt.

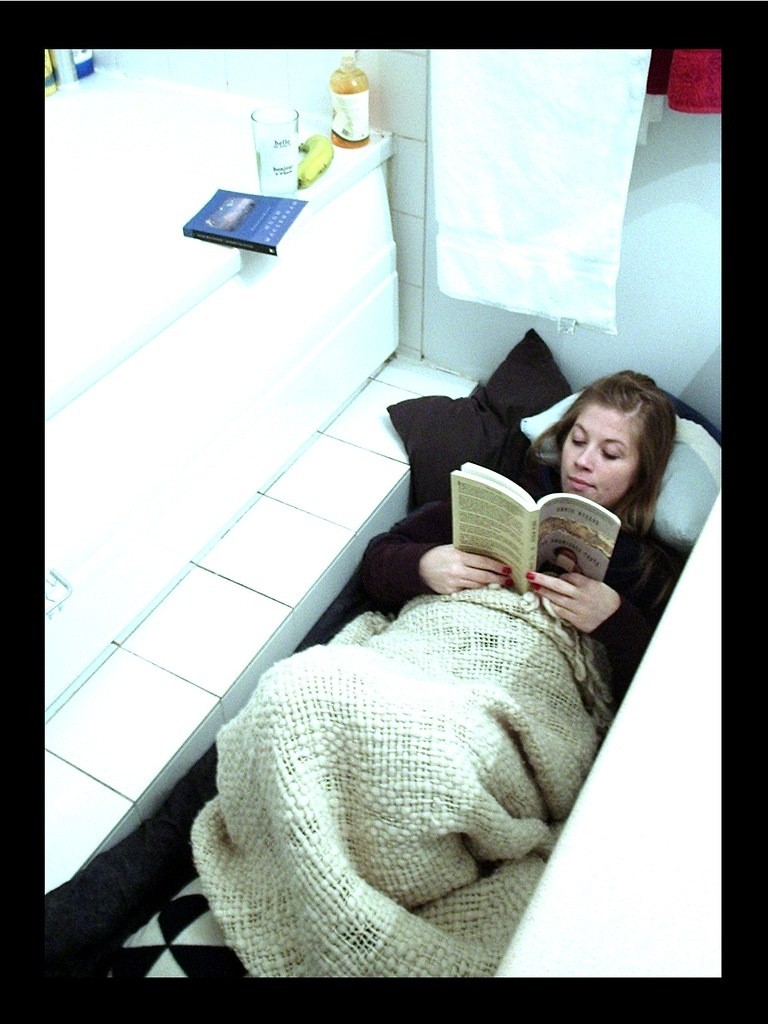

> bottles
[72,49,93,79]
[329,56,370,148]
[45,49,57,96]
[50,49,78,90]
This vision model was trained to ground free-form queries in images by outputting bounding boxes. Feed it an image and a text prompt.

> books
[182,189,308,258]
[451,461,622,598]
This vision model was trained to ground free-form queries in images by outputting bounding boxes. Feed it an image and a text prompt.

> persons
[45,370,688,977]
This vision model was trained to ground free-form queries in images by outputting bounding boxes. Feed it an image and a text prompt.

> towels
[429,49,664,336]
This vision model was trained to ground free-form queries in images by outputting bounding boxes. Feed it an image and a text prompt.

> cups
[252,107,299,197]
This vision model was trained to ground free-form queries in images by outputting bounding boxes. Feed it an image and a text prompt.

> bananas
[297,134,333,188]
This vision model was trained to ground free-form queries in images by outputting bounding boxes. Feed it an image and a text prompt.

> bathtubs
[45,353,726,976]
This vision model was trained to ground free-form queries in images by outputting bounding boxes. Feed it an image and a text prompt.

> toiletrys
[331,49,371,148]
